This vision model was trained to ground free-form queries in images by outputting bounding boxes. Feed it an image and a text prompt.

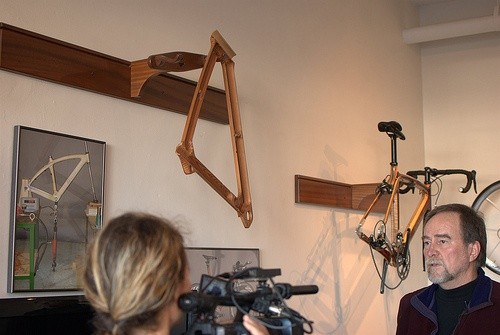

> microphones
[275,283,319,299]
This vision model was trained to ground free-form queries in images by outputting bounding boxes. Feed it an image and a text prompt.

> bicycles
[353,121,500,294]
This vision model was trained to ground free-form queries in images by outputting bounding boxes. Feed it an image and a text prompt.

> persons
[79,212,269,335]
[396,204,500,335]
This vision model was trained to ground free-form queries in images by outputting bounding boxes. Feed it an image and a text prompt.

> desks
[13,221,40,290]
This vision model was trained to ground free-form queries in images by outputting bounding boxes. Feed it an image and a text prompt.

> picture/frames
[184,246,260,299]
[6,125,106,293]
